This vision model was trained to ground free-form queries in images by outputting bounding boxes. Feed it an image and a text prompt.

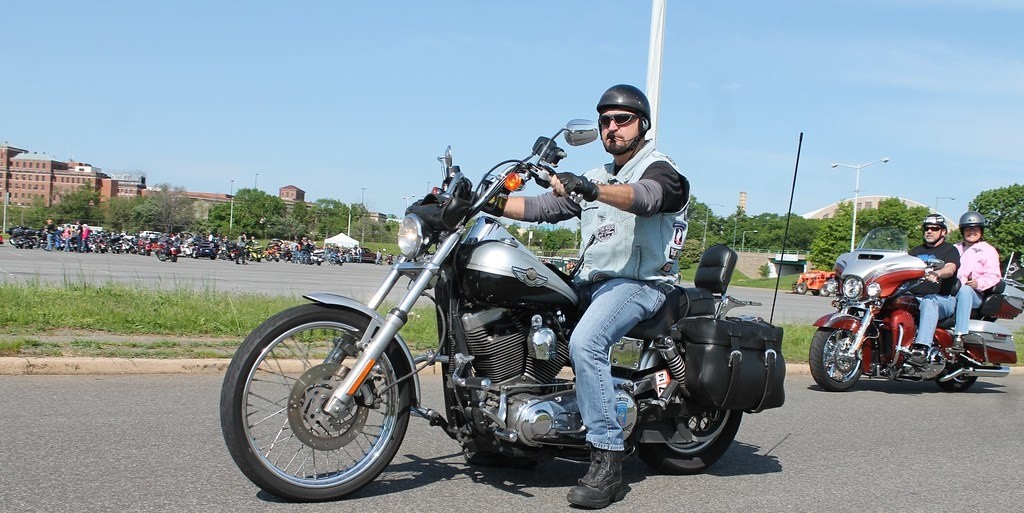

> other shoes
[907,345,931,366]
[953,335,964,349]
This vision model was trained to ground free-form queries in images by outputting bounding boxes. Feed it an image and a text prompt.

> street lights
[426,181,431,195]
[702,203,725,250]
[254,174,259,192]
[832,158,889,252]
[741,230,759,253]
[229,179,236,234]
[934,194,957,215]
[401,195,415,209]
[362,187,367,205]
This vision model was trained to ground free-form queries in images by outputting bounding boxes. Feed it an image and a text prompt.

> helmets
[596,84,651,130]
[922,214,948,234]
[959,212,985,238]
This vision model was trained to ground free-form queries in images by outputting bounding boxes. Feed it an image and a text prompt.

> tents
[324,233,359,249]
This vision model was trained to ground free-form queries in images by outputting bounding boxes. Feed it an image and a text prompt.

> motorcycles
[3,224,397,265]
[218,116,803,504]
[807,225,1024,393]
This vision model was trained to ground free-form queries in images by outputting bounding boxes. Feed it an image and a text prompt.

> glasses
[923,226,942,231]
[598,114,642,127]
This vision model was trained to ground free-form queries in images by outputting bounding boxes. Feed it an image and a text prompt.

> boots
[566,446,635,508]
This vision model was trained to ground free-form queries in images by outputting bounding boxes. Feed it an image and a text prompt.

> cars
[347,247,377,263]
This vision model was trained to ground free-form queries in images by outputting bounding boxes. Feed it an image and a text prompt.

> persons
[900,214,960,372]
[44,219,90,253]
[473,84,691,509]
[209,232,283,265]
[325,246,381,265]
[284,238,315,264]
[954,212,1001,350]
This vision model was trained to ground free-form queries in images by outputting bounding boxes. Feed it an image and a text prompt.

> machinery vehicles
[795,269,837,297]
